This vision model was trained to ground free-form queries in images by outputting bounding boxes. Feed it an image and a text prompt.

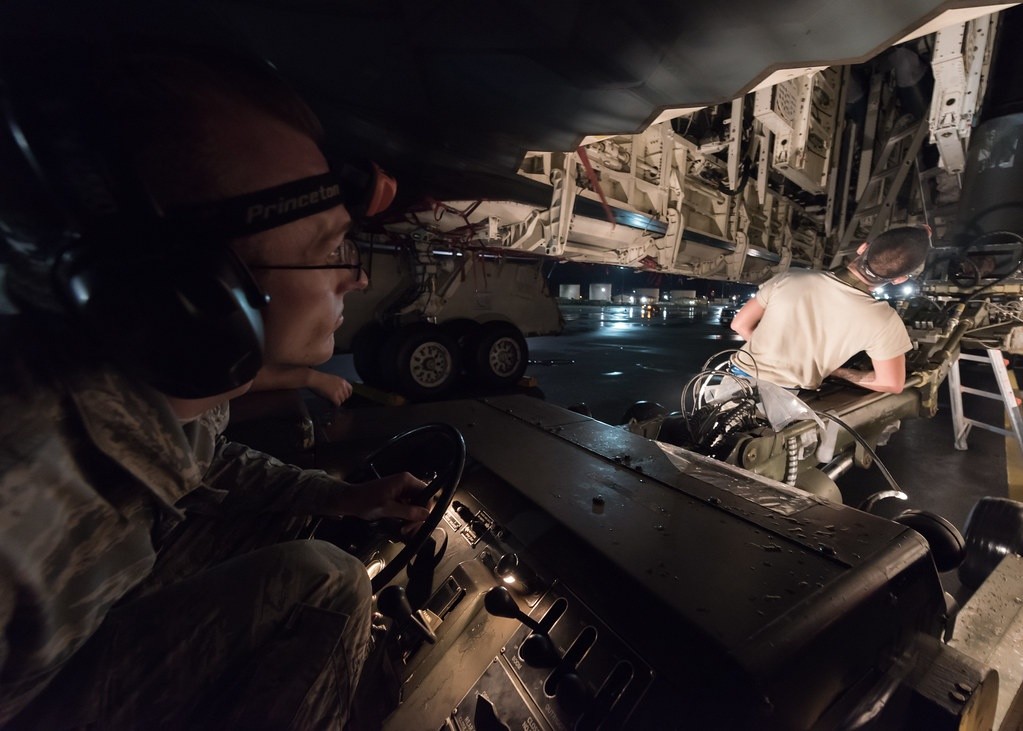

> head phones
[32,97,267,399]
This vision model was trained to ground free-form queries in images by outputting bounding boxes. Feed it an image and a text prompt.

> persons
[719,226,933,399]
[0,27,444,731]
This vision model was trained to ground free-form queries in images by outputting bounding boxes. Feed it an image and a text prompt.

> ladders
[830,106,1023,455]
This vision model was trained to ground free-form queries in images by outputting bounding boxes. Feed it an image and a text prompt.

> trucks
[342,251,565,398]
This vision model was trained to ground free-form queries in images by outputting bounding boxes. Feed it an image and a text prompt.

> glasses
[248,237,363,287]
